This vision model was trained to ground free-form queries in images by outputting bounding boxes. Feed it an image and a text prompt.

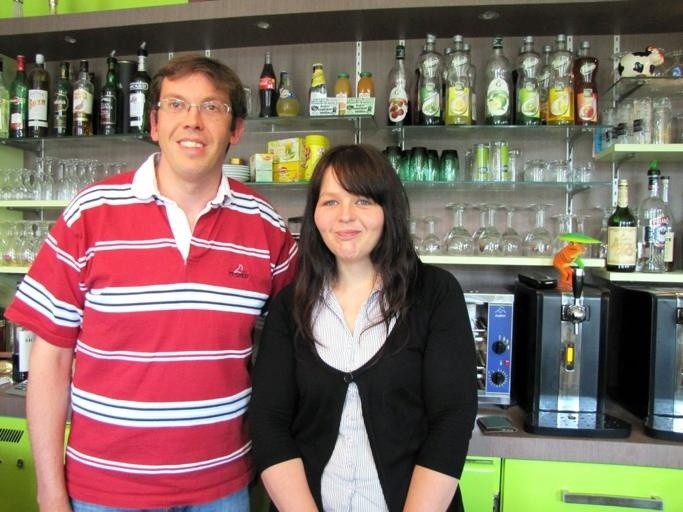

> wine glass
[406,202,555,257]
[0,155,129,267]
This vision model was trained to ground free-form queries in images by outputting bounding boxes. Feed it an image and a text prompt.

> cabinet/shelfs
[503,461,681,512]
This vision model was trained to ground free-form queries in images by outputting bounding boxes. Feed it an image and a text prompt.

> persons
[2,52,300,511]
[242,143,479,512]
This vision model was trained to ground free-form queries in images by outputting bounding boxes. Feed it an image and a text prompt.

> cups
[601,98,672,149]
[382,136,595,185]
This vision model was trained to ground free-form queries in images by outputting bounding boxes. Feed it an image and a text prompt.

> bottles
[607,179,637,273]
[637,168,669,273]
[660,176,675,271]
[10,280,38,381]
[0,33,601,136]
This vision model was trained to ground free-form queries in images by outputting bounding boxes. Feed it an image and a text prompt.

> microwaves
[464,290,516,407]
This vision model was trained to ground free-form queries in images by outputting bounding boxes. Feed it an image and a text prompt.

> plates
[221,164,251,182]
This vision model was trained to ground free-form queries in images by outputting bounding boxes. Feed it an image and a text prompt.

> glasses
[157,98,233,116]
